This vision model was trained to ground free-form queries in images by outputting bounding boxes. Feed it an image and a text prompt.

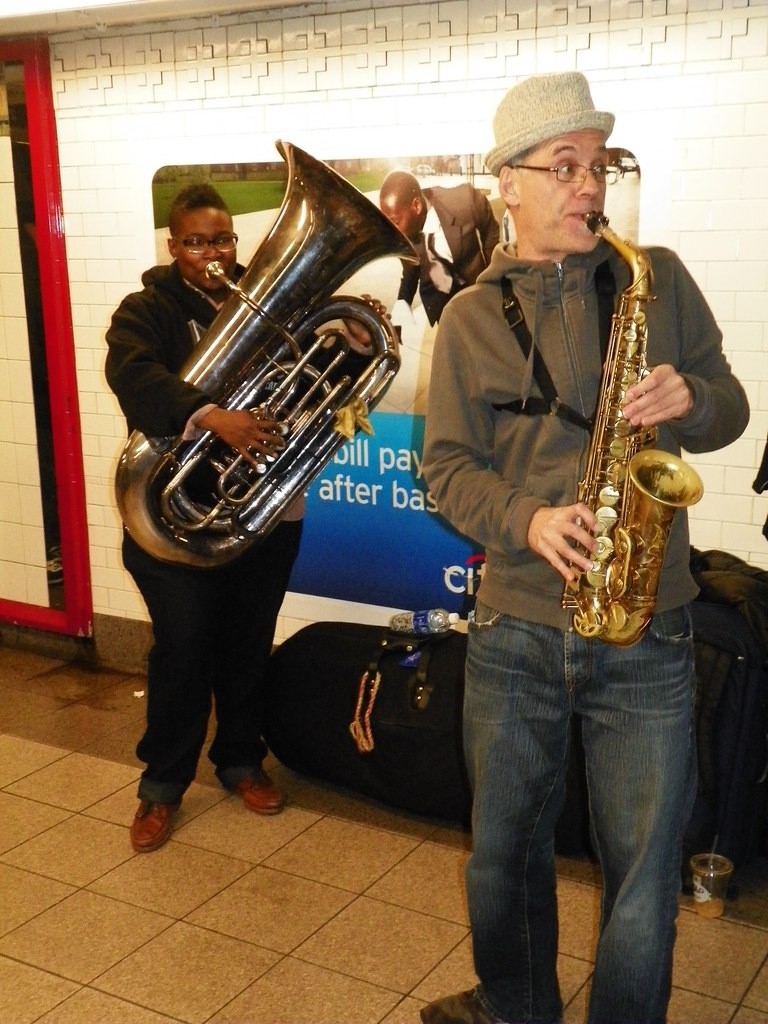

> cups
[689,852,733,918]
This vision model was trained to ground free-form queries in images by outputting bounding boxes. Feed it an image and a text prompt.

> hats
[484,72,614,178]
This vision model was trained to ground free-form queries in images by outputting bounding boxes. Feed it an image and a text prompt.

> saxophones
[561,209,704,652]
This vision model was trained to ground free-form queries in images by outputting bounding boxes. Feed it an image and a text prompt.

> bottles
[389,607,461,634]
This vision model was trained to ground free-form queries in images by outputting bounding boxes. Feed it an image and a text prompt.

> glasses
[171,231,238,254]
[507,164,626,184]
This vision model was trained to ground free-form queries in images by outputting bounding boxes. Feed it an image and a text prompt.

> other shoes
[420,988,508,1024]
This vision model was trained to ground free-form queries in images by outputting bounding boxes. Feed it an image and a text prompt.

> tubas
[112,135,423,572]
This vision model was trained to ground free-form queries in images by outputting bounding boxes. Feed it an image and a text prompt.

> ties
[427,234,454,276]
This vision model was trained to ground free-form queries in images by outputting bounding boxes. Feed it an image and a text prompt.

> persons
[420,73,752,1023]
[106,185,390,853]
[381,172,500,345]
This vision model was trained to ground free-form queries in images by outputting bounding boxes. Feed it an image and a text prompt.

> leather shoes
[210,757,286,815]
[129,793,184,853]
